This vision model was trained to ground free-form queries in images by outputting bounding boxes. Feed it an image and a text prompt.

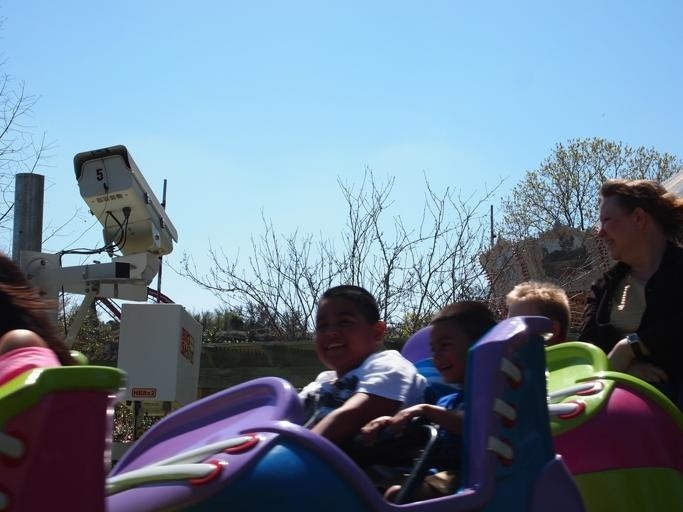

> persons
[505,280,571,347]
[0,250,79,391]
[575,175,683,413]
[360,299,497,506]
[297,283,429,493]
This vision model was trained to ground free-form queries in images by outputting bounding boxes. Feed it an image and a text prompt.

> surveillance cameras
[74,145,177,258]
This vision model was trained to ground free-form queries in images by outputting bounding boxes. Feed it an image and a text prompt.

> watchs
[626,332,645,362]
